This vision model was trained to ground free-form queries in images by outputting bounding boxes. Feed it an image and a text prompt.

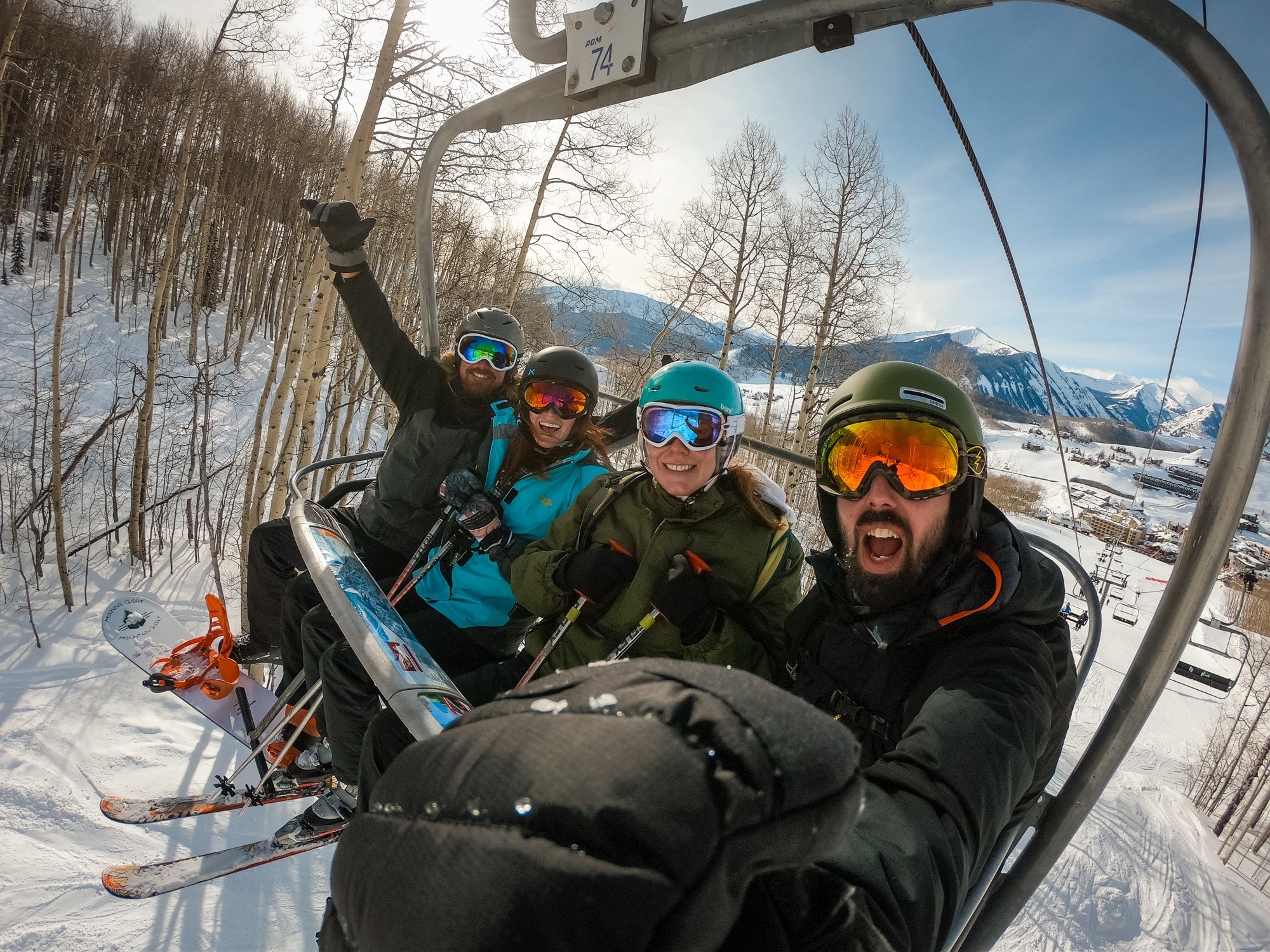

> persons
[1079,588,1083,597]
[1106,598,1109,605]
[1075,610,1088,630]
[1102,559,1105,562]
[356,360,804,815]
[722,362,1078,952]
[217,197,525,707]
[288,345,618,832]
[1099,557,1102,562]
[1062,602,1071,620]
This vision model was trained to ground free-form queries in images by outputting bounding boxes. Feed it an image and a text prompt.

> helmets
[455,308,524,362]
[517,346,599,416]
[813,361,988,535]
[637,360,744,457]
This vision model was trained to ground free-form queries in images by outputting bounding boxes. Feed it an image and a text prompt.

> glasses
[815,411,967,501]
[519,381,590,420]
[457,333,518,371]
[638,403,726,452]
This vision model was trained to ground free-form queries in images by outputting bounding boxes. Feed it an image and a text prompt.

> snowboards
[102,596,289,771]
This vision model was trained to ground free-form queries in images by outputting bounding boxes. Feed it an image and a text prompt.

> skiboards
[99,781,346,900]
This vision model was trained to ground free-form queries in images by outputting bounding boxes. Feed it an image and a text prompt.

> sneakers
[281,723,323,753]
[286,740,334,782]
[303,776,359,834]
[219,632,283,665]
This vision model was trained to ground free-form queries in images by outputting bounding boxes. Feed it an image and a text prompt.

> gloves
[456,492,520,562]
[299,198,377,272]
[554,541,638,610]
[331,655,866,952]
[438,468,483,508]
[657,554,714,644]
